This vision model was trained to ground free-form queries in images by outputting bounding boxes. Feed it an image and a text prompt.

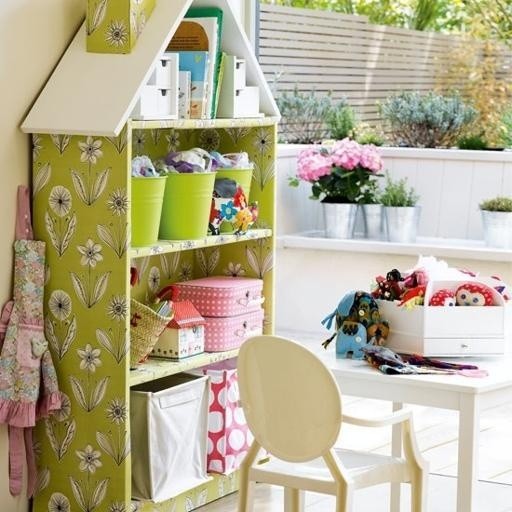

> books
[160,6,227,121]
[143,299,175,319]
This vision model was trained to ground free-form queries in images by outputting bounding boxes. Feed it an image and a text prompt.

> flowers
[289,137,384,200]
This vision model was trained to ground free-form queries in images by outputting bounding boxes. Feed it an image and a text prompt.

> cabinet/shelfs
[20,1,282,512]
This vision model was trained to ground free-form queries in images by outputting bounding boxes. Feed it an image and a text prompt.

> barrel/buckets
[171,171,218,243]
[218,165,260,239]
[130,173,169,247]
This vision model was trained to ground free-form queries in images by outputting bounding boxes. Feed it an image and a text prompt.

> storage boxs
[130,372,215,503]
[205,362,248,478]
[353,271,505,359]
[200,309,269,352]
[181,272,268,314]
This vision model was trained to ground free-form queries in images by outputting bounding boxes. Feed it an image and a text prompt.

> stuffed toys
[318,267,512,363]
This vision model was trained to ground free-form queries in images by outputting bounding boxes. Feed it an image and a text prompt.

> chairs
[232,335,425,512]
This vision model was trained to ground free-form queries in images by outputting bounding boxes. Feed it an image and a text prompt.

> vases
[322,202,356,238]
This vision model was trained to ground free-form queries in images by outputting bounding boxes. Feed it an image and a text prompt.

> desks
[291,338,507,511]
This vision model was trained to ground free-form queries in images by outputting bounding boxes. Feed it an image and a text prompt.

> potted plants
[381,177,419,239]
[480,198,512,241]
[361,178,385,237]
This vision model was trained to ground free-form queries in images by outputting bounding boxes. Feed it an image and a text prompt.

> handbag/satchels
[130,267,180,368]
